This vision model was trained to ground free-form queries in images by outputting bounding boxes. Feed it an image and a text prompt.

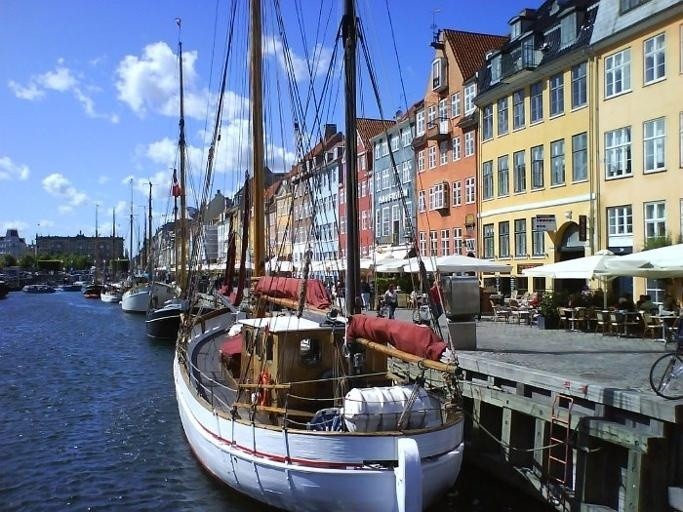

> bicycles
[648,325,682,402]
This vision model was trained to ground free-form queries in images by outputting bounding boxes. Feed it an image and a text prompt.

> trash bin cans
[397,293,407,308]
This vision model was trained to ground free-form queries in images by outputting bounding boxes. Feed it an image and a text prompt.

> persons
[636,294,646,308]
[429,280,443,320]
[639,294,657,310]
[359,275,370,309]
[564,293,577,309]
[408,287,418,310]
[383,283,398,319]
[617,297,633,310]
[333,279,345,312]
[579,285,590,297]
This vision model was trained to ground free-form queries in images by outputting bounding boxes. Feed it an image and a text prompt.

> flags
[172,170,180,198]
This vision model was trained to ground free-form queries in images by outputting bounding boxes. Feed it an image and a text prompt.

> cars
[142,268,250,291]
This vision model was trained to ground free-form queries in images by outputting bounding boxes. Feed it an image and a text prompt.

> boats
[22,280,103,298]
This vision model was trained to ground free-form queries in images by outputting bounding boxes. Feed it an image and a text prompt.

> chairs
[490,289,683,343]
